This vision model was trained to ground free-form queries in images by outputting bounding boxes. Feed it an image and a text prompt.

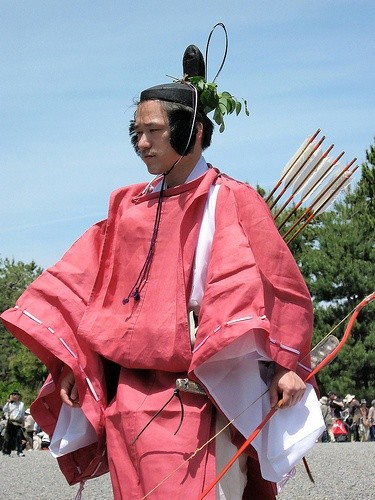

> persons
[3,390,26,457]
[315,392,375,443]
[1,22,327,500]
[0,405,51,452]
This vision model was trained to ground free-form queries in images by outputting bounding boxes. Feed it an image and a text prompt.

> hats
[319,395,327,403]
[139,23,250,133]
[345,394,355,403]
[9,390,21,395]
